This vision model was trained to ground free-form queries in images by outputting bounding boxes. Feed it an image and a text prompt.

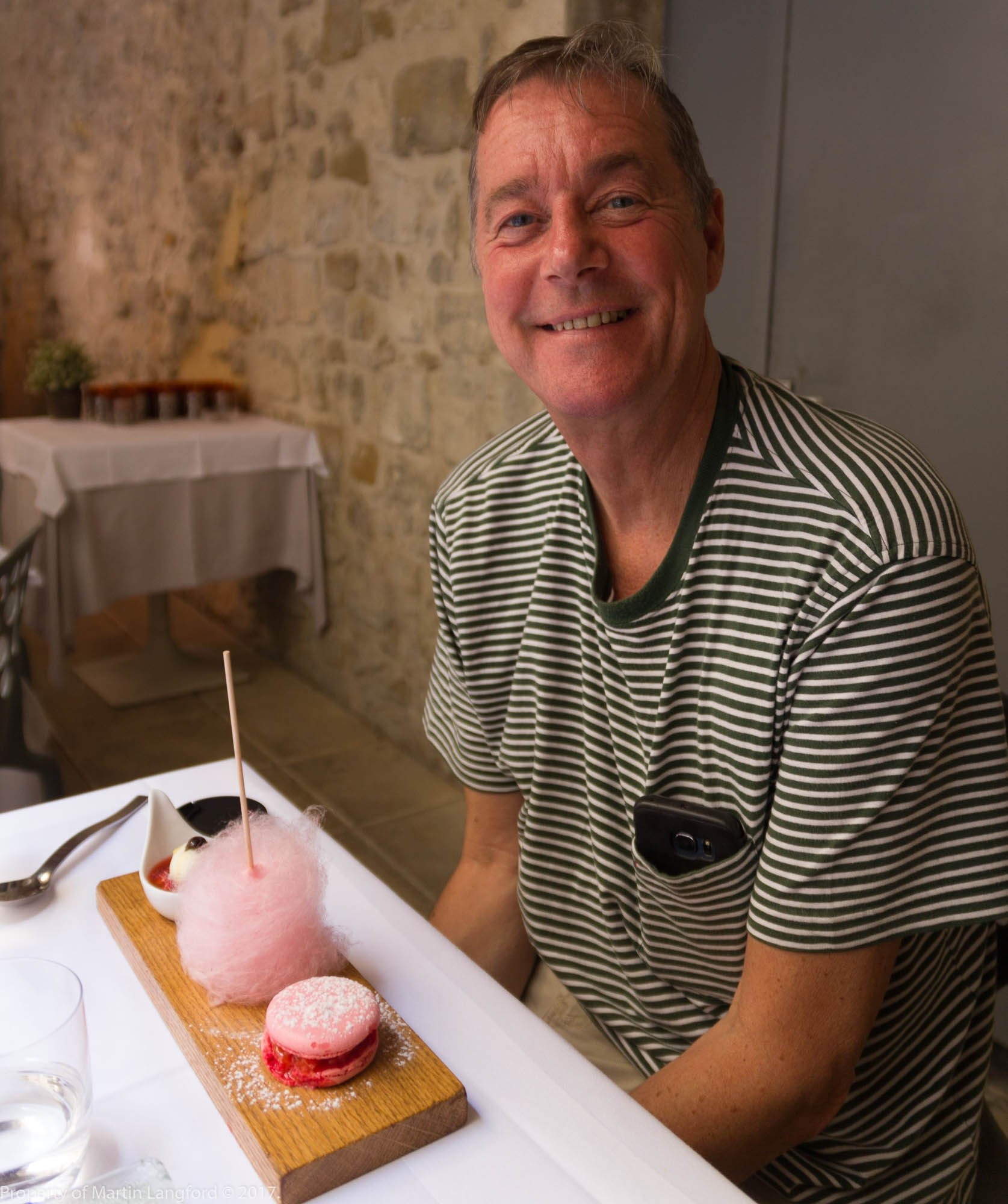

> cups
[1,957,92,1204]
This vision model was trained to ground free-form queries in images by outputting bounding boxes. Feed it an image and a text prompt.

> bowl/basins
[140,788,211,923]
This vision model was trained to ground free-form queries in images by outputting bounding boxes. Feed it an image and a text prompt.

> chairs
[0,522,61,804]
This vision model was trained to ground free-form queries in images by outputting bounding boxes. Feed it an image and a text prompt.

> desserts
[145,836,217,892]
[260,976,382,1086]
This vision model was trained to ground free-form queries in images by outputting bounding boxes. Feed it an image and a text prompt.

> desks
[0,756,754,1204]
[0,416,331,707]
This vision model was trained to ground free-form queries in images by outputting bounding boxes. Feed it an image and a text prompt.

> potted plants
[22,335,98,418]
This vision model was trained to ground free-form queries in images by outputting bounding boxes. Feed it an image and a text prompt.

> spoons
[0,795,149,904]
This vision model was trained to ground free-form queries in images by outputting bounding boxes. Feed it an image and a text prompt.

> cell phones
[632,795,749,876]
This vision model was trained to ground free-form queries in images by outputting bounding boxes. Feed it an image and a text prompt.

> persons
[418,37,1006,1204]
